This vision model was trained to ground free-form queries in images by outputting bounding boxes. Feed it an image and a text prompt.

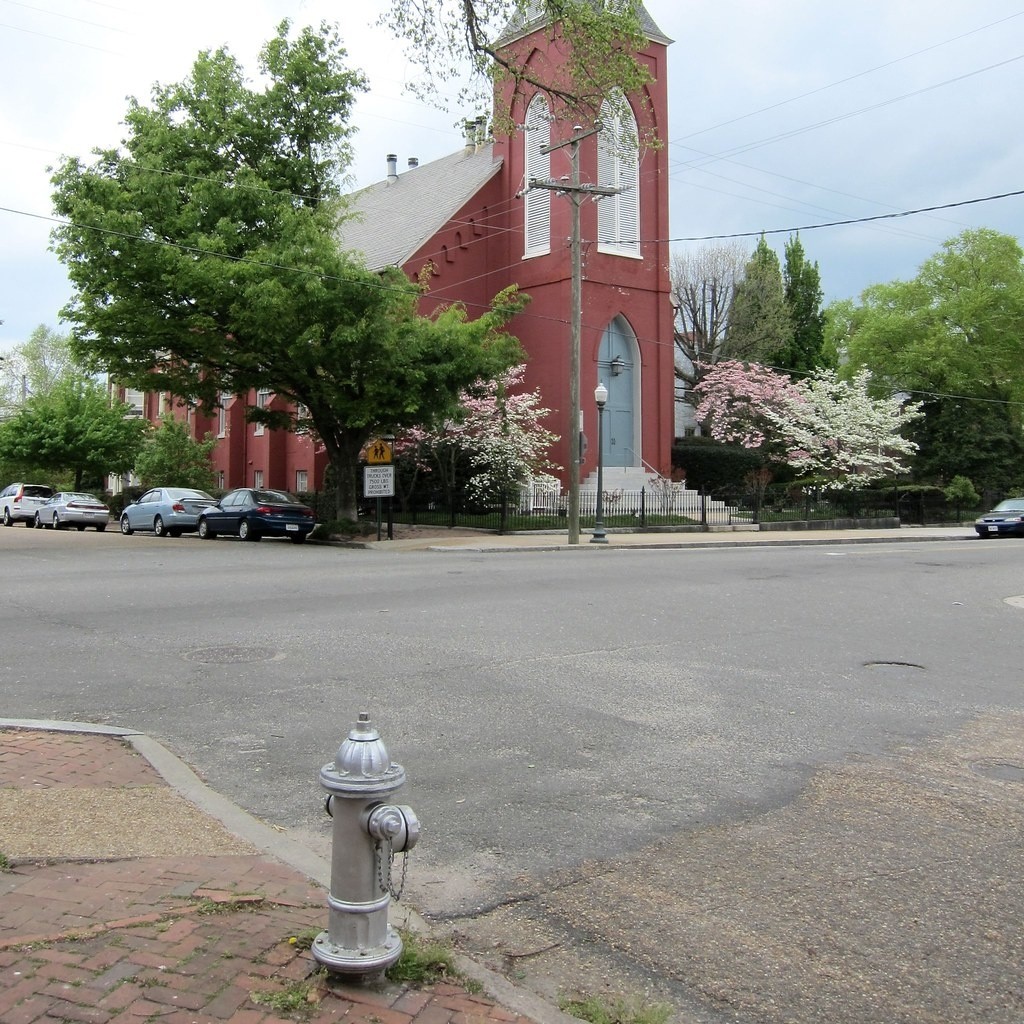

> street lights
[589,380,611,543]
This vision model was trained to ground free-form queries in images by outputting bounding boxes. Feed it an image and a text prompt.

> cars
[975,496,1024,538]
[196,488,316,544]
[120,487,219,537]
[34,492,110,532]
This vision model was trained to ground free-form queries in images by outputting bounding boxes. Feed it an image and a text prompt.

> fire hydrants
[311,711,422,987]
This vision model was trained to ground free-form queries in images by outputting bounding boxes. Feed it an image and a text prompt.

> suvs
[0,482,53,527]
